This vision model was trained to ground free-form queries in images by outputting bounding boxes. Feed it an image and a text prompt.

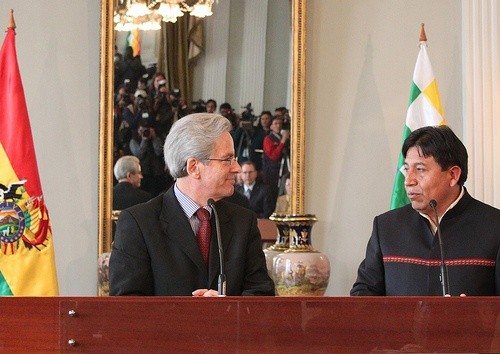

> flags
[390,40,448,211]
[0,30,59,297]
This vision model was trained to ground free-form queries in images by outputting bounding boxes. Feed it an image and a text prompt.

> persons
[186,98,291,177]
[229,161,272,218]
[350,125,500,296]
[109,112,275,298]
[113,156,153,210]
[274,173,291,214]
[113,45,187,185]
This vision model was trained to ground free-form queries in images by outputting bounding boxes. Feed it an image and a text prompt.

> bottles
[262,215,291,282]
[97,210,124,297]
[273,214,330,298]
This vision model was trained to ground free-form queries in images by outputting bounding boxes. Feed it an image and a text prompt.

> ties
[246,188,251,199]
[194,207,212,264]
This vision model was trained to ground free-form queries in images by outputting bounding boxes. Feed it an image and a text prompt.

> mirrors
[99,0,307,295]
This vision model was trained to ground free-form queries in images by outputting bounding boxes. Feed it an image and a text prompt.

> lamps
[114,1,218,30]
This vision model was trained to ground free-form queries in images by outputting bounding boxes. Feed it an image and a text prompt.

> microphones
[428,199,450,296]
[207,198,226,297]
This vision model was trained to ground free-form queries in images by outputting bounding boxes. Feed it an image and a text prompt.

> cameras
[143,129,151,138]
[170,89,180,106]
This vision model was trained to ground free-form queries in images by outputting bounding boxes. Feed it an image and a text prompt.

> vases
[272,214,331,296]
[263,215,290,281]
[98,210,124,297]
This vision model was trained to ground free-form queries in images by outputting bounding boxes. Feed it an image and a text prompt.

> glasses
[184,157,238,166]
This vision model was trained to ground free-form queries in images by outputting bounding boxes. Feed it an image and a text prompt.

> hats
[134,90,147,98]
